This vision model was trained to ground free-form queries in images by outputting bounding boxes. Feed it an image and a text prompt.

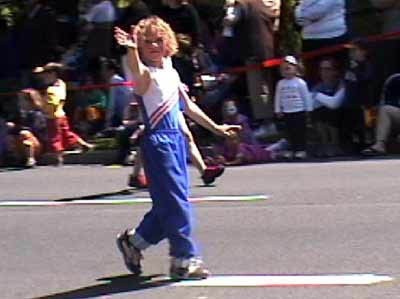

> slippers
[360,147,386,157]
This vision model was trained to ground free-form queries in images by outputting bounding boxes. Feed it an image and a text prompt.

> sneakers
[116,229,141,274]
[201,165,224,184]
[168,257,210,279]
[129,174,148,187]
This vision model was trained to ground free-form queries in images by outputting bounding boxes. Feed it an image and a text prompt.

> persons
[0,0,400,190]
[115,14,243,281]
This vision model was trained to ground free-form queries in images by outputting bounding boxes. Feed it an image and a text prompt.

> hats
[283,55,299,66]
[343,37,369,51]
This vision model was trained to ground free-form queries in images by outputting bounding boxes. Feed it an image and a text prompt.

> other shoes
[280,150,306,159]
[316,145,340,157]
